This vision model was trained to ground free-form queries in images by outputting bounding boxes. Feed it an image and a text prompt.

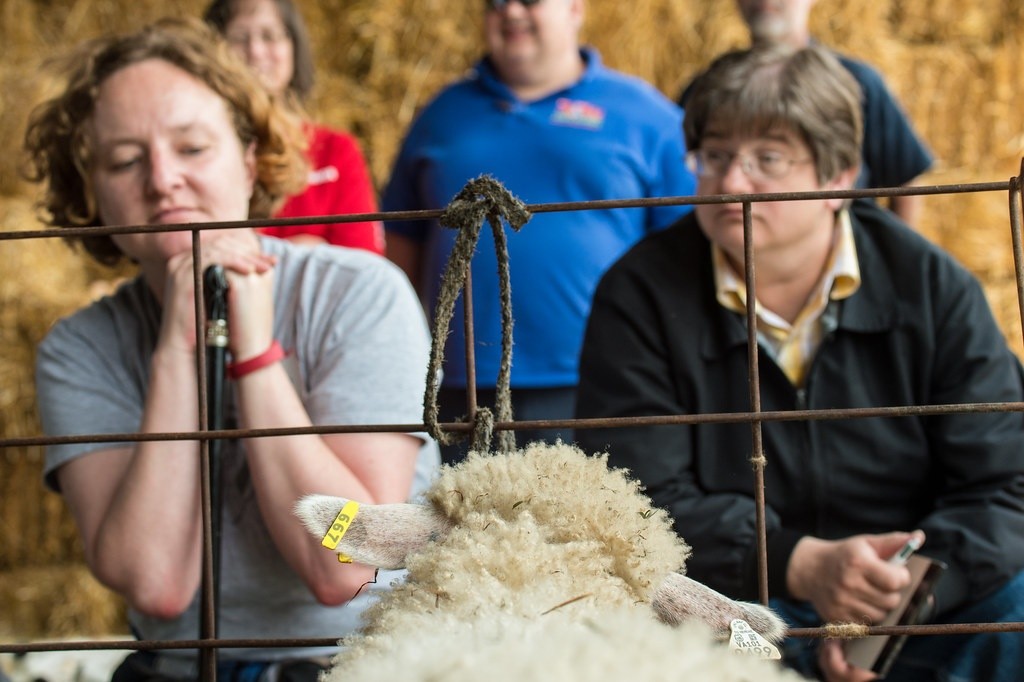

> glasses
[489,0,540,14]
[682,147,815,179]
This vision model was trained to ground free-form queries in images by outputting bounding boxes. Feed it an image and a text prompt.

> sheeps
[294,439,806,682]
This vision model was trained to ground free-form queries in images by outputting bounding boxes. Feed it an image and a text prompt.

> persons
[575,48,1024,682]
[202,0,388,259]
[20,15,445,682]
[382,0,702,458]
[678,0,931,228]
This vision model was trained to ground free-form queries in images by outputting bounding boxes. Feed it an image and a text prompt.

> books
[842,552,947,675]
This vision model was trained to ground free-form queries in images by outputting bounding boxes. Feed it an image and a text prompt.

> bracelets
[223,341,283,379]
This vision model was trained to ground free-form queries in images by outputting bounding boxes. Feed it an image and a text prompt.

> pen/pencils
[888,537,919,566]
